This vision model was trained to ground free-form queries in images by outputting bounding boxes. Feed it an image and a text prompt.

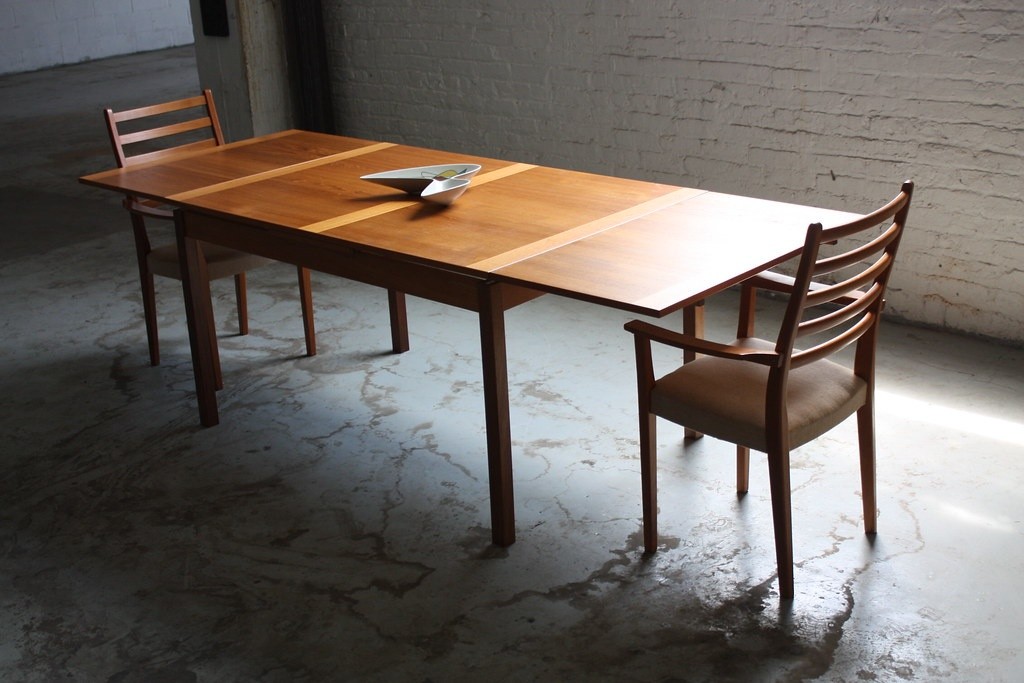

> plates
[359,163,482,204]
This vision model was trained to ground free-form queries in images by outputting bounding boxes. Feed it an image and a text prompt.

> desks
[77,129,865,550]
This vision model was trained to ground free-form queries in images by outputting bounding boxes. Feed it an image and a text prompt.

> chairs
[622,180,914,600]
[103,88,317,365]
